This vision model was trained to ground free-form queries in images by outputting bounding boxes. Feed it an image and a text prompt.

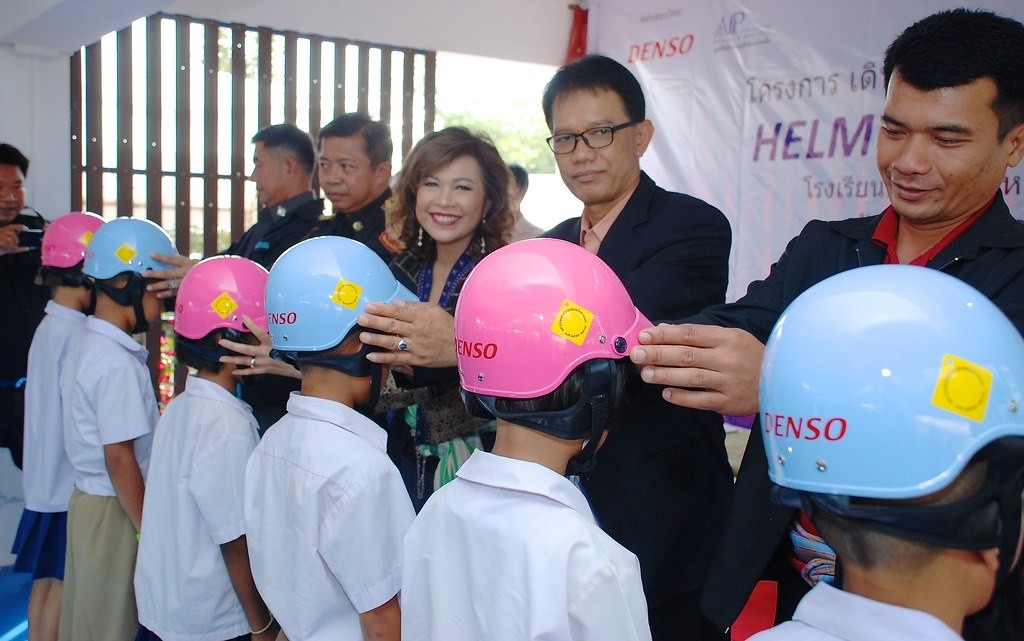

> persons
[739,263,1023,641]
[145,111,402,437]
[59,219,179,641]
[158,123,323,422]
[631,8,1024,641]
[355,53,734,641]
[135,255,279,641]
[0,141,49,471]
[11,213,107,641]
[402,237,656,641]
[246,237,421,640]
[510,163,544,242]
[217,126,511,515]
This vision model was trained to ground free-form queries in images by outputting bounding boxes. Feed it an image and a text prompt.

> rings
[397,336,407,351]
[250,357,256,368]
[168,279,177,292]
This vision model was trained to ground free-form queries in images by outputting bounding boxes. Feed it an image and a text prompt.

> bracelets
[249,613,273,634]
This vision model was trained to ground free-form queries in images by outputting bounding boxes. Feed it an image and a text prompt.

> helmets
[42,211,106,266]
[172,254,269,339]
[759,265,1024,498]
[82,217,179,281]
[264,237,420,352]
[454,238,654,397]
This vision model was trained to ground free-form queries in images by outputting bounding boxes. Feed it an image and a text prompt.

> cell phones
[17,228,44,247]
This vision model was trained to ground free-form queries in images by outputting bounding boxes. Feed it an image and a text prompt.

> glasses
[546,119,636,154]
